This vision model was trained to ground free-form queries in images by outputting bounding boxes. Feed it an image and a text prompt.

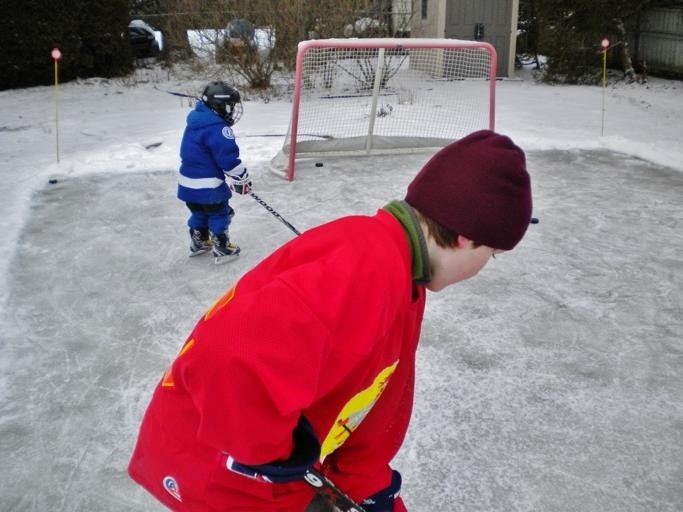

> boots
[188,228,213,251]
[212,235,240,257]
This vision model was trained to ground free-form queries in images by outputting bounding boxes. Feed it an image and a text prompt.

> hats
[406,130,532,250]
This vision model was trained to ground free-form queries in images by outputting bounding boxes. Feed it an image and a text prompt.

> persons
[124,129,533,512]
[174,79,255,259]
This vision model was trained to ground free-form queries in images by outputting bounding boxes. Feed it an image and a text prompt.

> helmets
[202,80,243,126]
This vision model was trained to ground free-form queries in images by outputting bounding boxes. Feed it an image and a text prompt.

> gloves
[227,416,323,486]
[333,470,402,512]
[225,167,252,194]
